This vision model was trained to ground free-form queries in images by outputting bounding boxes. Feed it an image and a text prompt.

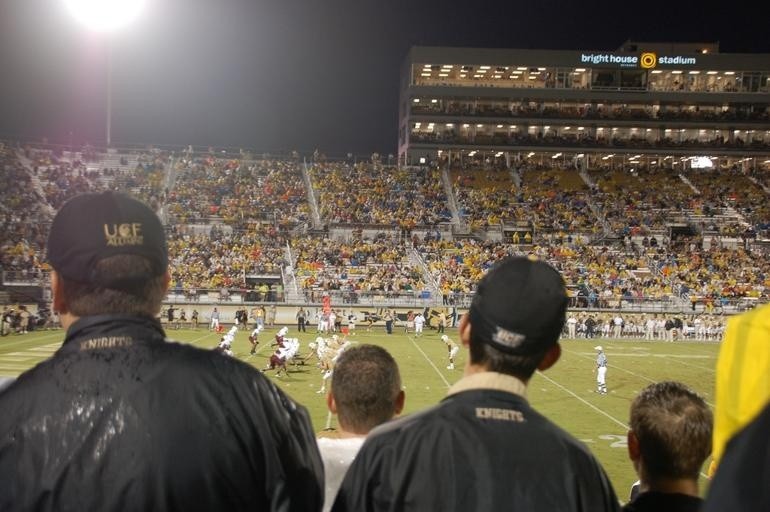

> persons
[330,256,619,512]
[409,80,769,151]
[1,192,324,510]
[218,325,343,393]
[700,401,769,507]
[593,347,608,394]
[2,139,447,338]
[441,335,459,370]
[310,345,404,511]
[614,384,713,510]
[407,154,770,344]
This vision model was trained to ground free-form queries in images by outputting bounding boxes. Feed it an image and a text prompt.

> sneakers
[447,363,456,369]
[260,369,282,378]
[595,388,608,395]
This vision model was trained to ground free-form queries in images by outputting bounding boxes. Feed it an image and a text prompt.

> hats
[41,187,169,284]
[466,248,570,356]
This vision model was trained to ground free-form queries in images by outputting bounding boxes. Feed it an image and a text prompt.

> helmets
[441,334,448,342]
[223,324,351,357]
[594,346,604,352]
